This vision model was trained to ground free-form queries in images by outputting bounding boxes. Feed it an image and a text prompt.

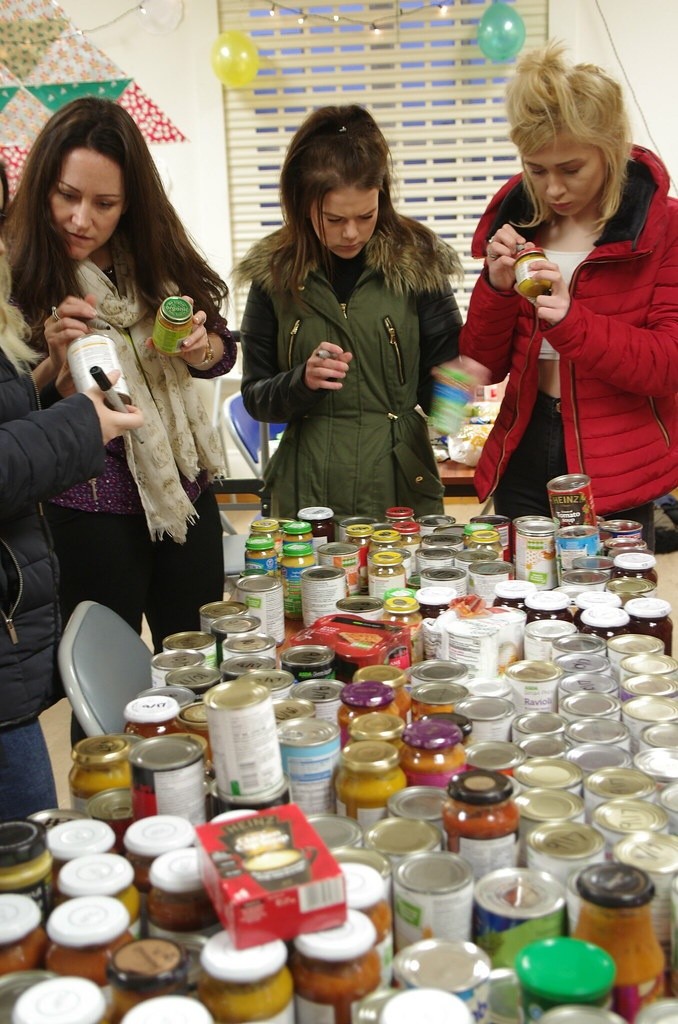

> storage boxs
[285,612,414,679]
[193,797,354,954]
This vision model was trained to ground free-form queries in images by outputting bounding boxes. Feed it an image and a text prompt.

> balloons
[477,1,527,59]
[212,31,258,89]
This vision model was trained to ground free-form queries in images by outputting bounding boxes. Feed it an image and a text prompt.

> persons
[459,33,678,553]
[235,103,466,520]
[0,97,237,791]
[0,160,143,822]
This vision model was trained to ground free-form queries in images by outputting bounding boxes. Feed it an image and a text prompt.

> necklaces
[90,251,114,274]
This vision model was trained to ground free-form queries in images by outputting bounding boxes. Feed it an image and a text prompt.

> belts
[533,394,563,419]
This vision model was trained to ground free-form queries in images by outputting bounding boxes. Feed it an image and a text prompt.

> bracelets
[182,337,214,369]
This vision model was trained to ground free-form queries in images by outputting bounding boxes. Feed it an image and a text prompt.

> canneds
[67,334,132,411]
[0,473,678,1024]
[151,297,193,356]
[514,246,551,297]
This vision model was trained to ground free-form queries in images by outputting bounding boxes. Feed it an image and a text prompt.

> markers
[514,241,524,249]
[316,349,337,361]
[72,314,109,329]
[89,366,145,444]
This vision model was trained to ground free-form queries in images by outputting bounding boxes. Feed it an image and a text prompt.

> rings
[488,236,495,245]
[51,306,60,321]
[488,246,497,258]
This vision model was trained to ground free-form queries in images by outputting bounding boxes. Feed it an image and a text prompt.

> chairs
[52,596,161,746]
[221,390,287,479]
